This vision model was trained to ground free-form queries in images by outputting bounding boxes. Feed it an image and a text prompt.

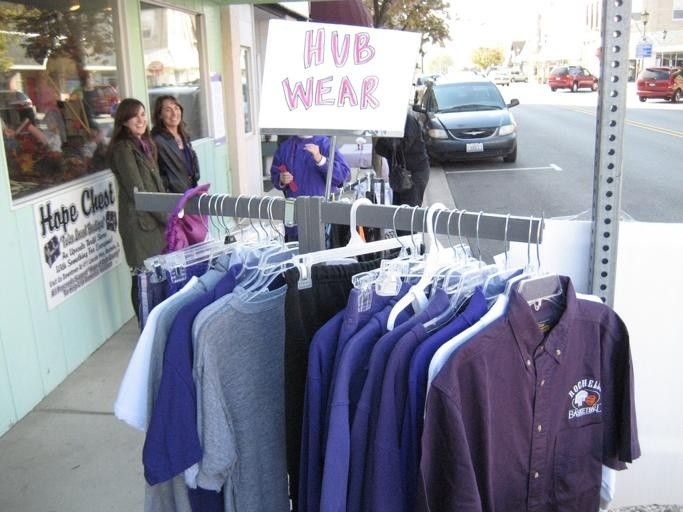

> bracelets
[315,155,326,167]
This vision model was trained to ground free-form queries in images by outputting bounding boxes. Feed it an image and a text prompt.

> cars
[548,65,599,92]
[412,76,435,92]
[0,90,36,125]
[636,66,683,103]
[412,75,520,167]
[464,66,529,86]
[68,84,119,116]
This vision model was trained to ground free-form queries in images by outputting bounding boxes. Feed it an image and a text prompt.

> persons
[149,96,200,192]
[270,135,351,249]
[1,90,111,174]
[107,98,169,319]
[375,114,430,207]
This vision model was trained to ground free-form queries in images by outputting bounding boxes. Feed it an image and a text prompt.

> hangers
[140,191,301,314]
[292,197,604,350]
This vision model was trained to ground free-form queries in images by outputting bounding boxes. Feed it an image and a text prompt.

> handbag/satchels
[389,165,413,192]
[163,182,210,252]
